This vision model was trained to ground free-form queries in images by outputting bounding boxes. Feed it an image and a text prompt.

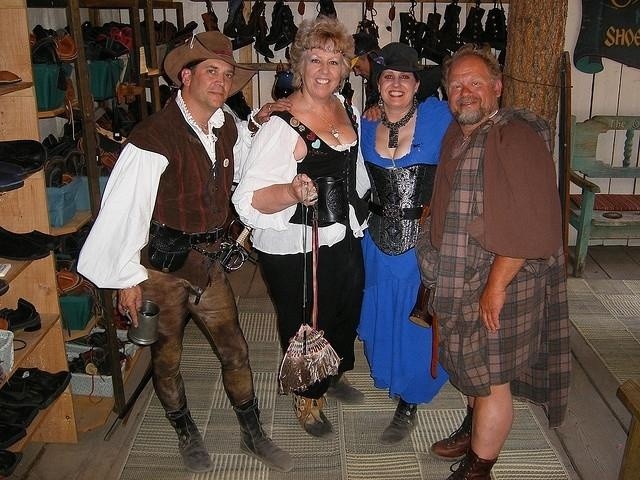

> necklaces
[381,98,418,149]
[329,125,340,138]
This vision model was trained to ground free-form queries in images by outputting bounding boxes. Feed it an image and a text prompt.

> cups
[409,285,434,329]
[125,300,162,345]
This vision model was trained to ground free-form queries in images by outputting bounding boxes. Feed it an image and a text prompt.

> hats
[164,29,259,100]
[366,42,442,101]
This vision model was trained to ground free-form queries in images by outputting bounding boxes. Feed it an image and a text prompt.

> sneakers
[0,298,42,334]
[1,367,73,480]
[0,138,49,193]
[326,375,364,405]
[0,224,64,262]
[292,393,339,440]
[459,6,508,50]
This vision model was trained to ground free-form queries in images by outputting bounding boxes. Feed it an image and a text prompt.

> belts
[149,220,228,246]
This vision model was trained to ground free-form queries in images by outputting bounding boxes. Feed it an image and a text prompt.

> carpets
[106,297,582,480]
[567,278,640,388]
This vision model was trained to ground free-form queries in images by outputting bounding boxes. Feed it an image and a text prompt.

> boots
[165,398,214,475]
[421,3,467,66]
[378,397,419,446]
[430,405,473,462]
[222,0,255,50]
[264,0,299,51]
[248,0,275,59]
[399,10,426,63]
[445,448,498,479]
[232,395,297,474]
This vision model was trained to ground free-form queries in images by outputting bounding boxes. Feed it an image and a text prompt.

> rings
[268,104,273,111]
[124,308,132,324]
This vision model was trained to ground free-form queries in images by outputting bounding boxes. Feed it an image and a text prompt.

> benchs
[569,116,640,277]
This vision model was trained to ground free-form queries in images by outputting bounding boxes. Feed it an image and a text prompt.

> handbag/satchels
[276,323,342,392]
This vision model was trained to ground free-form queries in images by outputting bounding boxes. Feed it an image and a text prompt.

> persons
[356,41,457,447]
[349,33,383,110]
[75,31,295,474]
[361,42,572,480]
[230,12,366,439]
